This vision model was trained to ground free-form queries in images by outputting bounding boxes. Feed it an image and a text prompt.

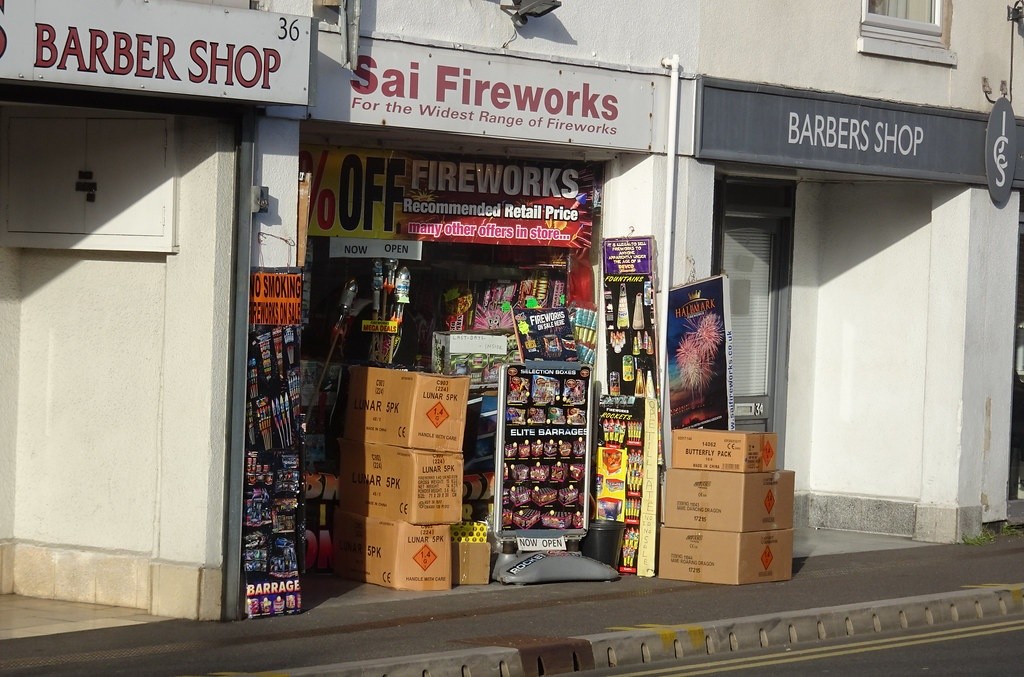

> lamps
[513,0,562,28]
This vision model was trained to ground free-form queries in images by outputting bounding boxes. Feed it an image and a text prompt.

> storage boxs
[328,365,492,590]
[432,330,509,383]
[658,427,796,585]
